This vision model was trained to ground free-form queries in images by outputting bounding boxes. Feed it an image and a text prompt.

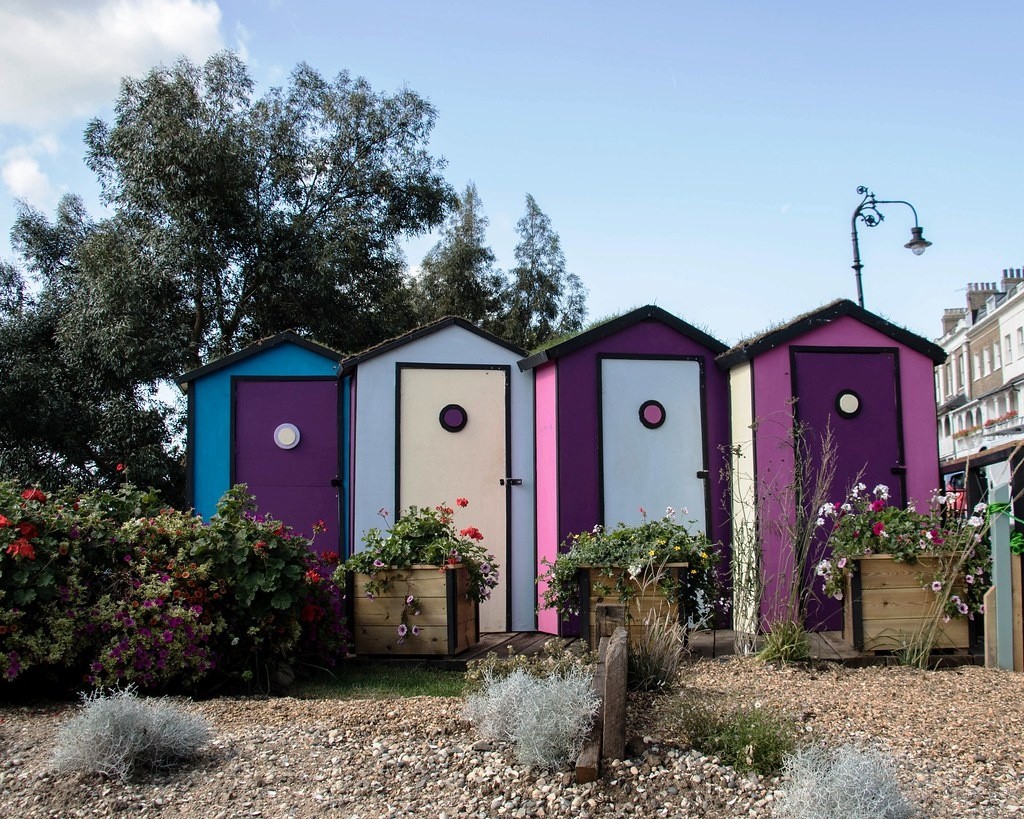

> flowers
[533,505,733,631]
[330,497,501,646]
[814,483,993,623]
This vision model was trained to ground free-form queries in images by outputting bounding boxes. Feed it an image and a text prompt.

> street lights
[850,184,933,309]
